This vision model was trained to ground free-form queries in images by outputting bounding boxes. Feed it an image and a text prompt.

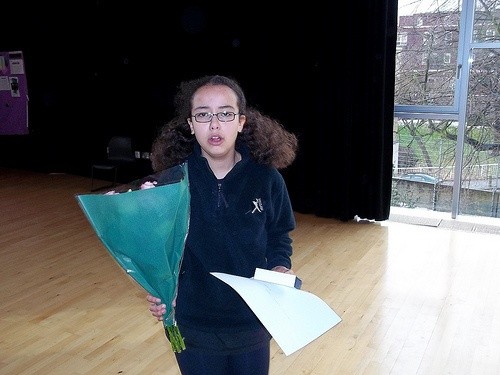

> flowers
[75,161,192,353]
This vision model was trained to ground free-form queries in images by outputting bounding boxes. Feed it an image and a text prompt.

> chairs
[91,137,132,184]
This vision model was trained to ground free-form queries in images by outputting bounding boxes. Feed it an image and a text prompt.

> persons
[146,75,297,375]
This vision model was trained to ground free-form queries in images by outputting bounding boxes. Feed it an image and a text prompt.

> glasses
[191,112,239,122]
